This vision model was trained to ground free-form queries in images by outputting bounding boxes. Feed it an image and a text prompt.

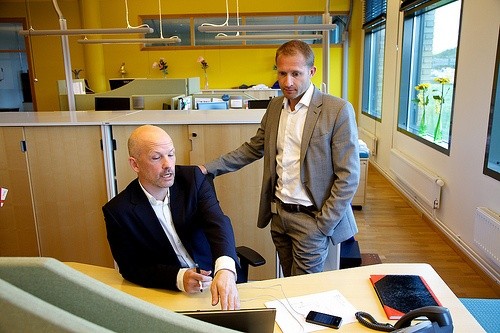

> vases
[434,113,444,143]
[163,74,167,79]
[419,115,426,136]
[203,73,209,90]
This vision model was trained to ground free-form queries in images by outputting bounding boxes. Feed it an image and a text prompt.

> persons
[200,40,361,278]
[101,125,241,311]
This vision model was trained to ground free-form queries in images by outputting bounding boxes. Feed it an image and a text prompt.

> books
[369,274,443,320]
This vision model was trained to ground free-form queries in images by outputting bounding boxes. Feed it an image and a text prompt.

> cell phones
[305,311,342,329]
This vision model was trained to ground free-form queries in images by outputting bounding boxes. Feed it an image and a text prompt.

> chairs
[235,244,268,284]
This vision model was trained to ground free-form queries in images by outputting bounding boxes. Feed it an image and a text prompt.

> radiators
[388,148,445,213]
[359,126,378,160]
[472,205,500,269]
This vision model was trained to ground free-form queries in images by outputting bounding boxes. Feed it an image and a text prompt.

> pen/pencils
[195,263,203,293]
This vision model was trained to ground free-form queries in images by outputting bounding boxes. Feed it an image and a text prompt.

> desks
[53,262,487,333]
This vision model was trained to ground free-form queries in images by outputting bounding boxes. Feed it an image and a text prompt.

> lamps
[198,0,338,34]
[75,0,183,45]
[214,0,326,40]
[17,0,155,36]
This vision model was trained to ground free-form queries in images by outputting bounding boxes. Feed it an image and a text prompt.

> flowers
[152,57,168,78]
[432,72,454,120]
[412,83,431,113]
[194,56,210,89]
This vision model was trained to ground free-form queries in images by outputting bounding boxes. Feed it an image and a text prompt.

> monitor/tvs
[109,79,133,90]
[248,100,270,109]
[95,97,130,110]
[197,102,228,109]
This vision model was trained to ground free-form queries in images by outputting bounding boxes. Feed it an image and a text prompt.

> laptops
[176,308,276,333]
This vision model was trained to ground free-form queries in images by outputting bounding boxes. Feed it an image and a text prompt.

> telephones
[388,306,454,333]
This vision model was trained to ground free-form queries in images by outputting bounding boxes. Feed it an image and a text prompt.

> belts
[274,195,317,219]
